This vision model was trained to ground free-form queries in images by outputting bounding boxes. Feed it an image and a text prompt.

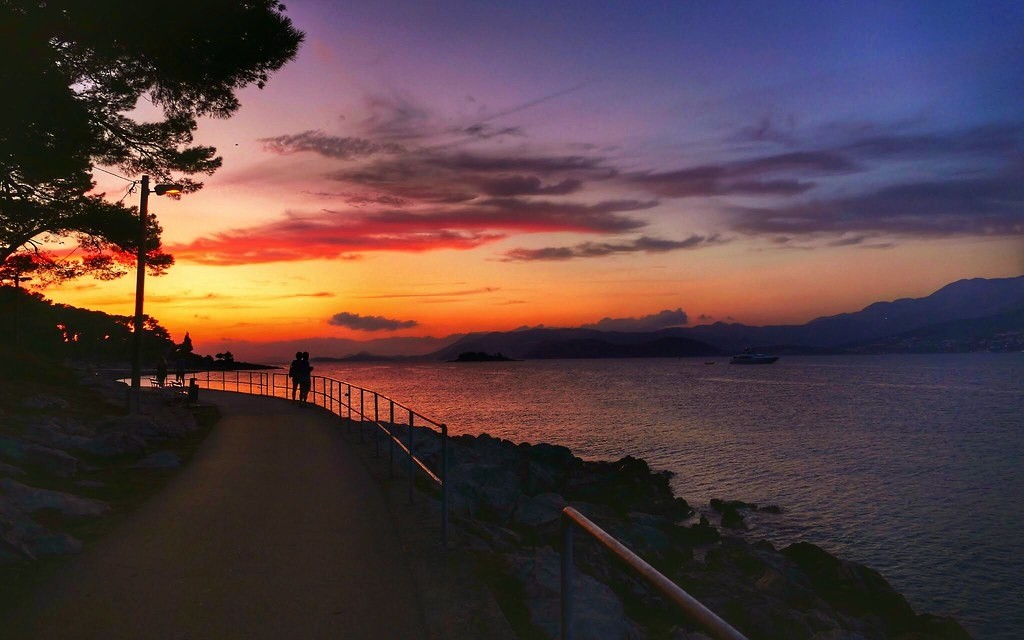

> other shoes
[303,403,312,408]
[298,401,303,406]
[292,401,299,404]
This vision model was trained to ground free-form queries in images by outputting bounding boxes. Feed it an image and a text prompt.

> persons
[290,351,314,408]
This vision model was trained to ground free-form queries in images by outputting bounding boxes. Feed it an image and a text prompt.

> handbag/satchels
[289,365,294,377]
